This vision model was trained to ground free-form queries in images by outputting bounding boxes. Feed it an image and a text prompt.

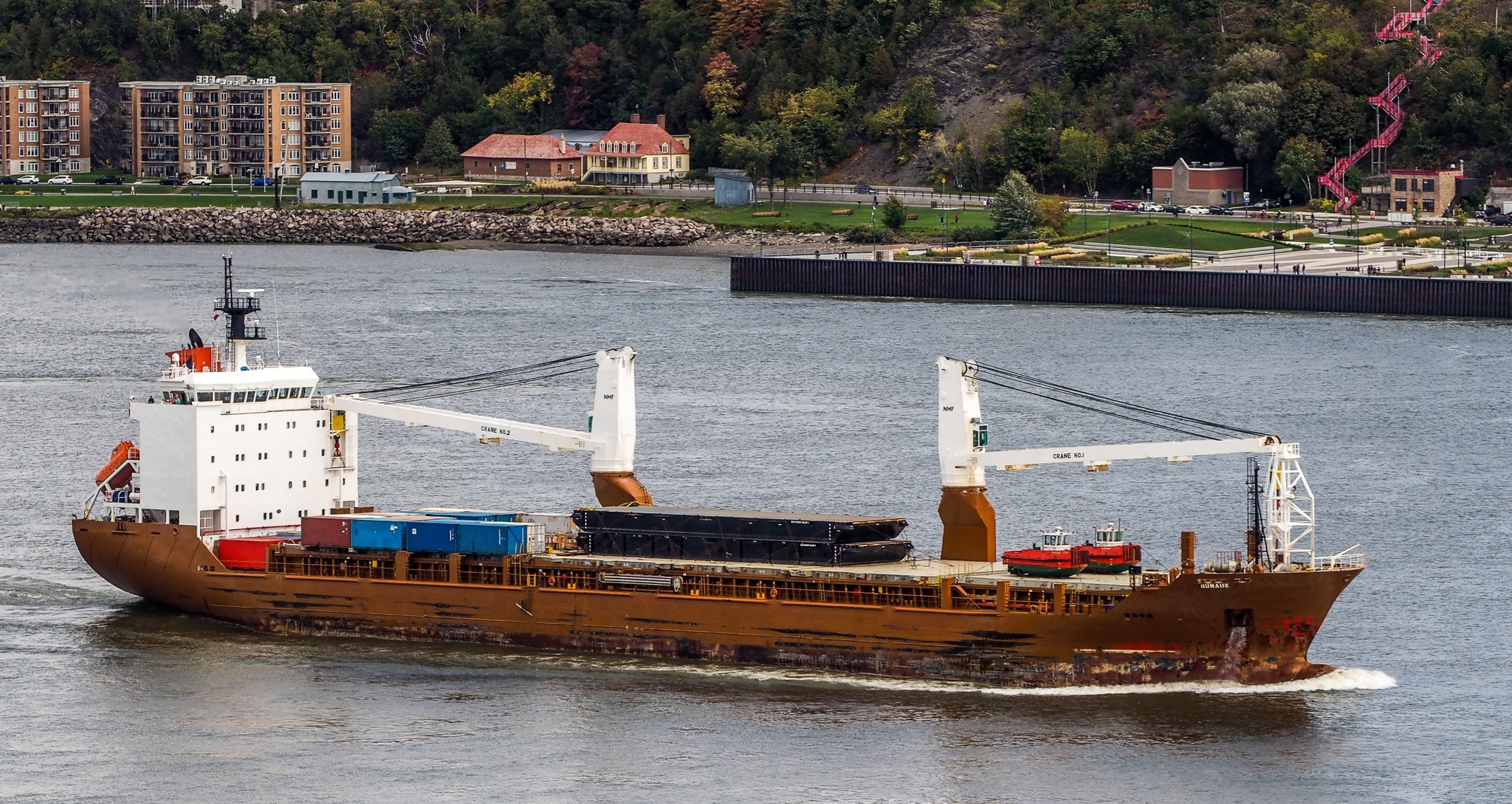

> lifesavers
[291,390,296,395]
[1030,605,1037,612]
[548,577,556,586]
[106,492,113,500]
[770,588,778,598]
[1034,258,1041,265]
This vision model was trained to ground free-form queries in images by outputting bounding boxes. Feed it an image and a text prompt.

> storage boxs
[301,509,546,555]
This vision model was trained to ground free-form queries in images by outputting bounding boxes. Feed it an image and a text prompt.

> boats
[71,256,1366,690]
[1075,519,1142,575]
[95,440,141,499]
[1000,526,1090,579]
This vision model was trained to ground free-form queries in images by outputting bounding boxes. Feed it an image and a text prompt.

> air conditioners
[196,76,202,83]
[233,81,239,85]
[227,80,232,85]
[270,76,276,84]
[220,80,226,85]
[257,78,263,85]
[249,80,255,84]
[263,79,269,84]
[209,76,216,83]
[203,76,209,83]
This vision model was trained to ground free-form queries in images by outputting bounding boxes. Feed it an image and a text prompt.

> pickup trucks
[94,174,125,185]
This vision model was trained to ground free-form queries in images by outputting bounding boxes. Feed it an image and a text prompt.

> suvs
[854,185,877,195]
[17,175,39,185]
[1137,201,1164,212]
[1110,200,1135,211]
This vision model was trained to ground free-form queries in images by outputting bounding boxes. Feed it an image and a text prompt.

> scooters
[1274,215,1283,221]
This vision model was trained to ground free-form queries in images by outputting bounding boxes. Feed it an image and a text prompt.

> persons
[983,199,993,210]
[1066,205,1070,213]
[0,203,5,211]
[1276,210,1281,220]
[553,535,557,549]
[1080,203,1084,211]
[1347,228,1350,238]
[1403,257,1406,265]
[1504,270,1510,278]
[1292,264,1306,274]
[1261,210,1266,220]
[131,185,136,195]
[955,213,959,223]
[1276,263,1279,274]
[1311,212,1315,225]
[1368,265,1380,276]
[170,394,180,405]
[814,249,819,258]
[148,396,154,403]
[1338,217,1342,227]
[1488,234,1497,245]
[1259,264,1262,272]
[625,186,633,195]
[1135,204,1139,212]
[873,195,877,205]
[1487,267,1490,274]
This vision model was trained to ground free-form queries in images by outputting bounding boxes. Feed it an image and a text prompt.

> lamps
[1424,180,1428,184]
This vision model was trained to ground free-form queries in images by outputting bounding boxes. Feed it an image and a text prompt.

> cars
[159,177,184,186]
[250,177,273,187]
[1209,205,1234,216]
[48,175,73,185]
[0,177,22,186]
[1185,206,1210,216]
[1255,198,1276,209]
[1476,210,1512,227]
[1163,205,1186,213]
[187,176,213,185]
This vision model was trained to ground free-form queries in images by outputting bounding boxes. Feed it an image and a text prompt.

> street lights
[1288,200,1293,230]
